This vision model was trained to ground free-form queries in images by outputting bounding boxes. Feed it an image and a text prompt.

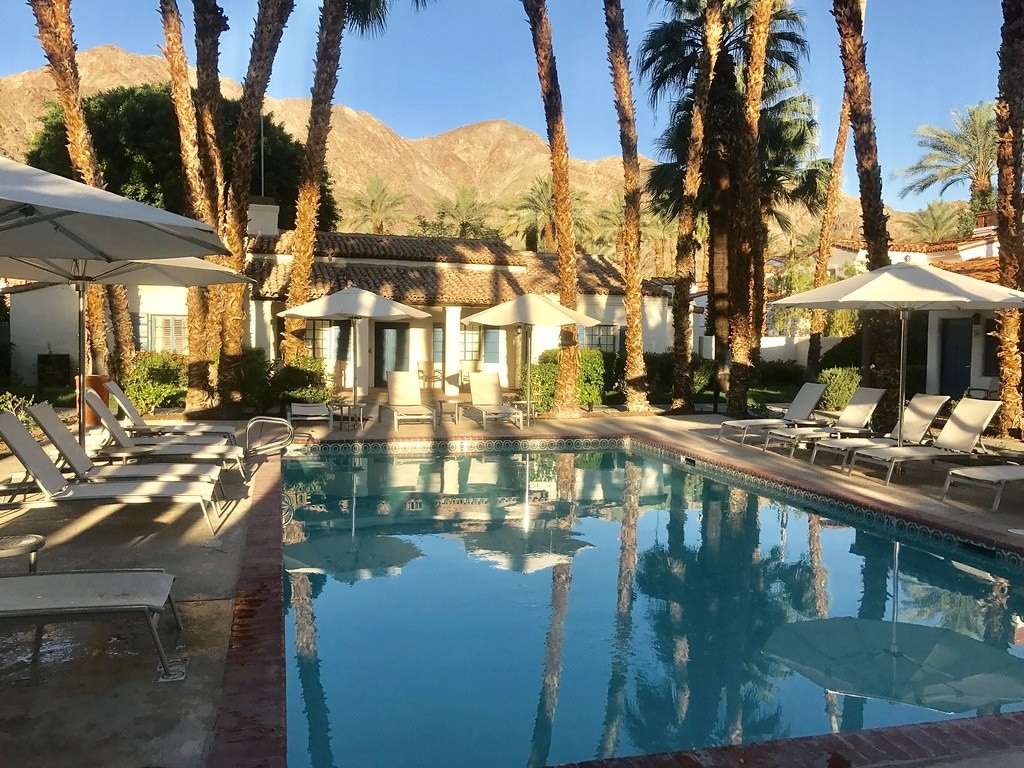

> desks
[438,400,463,426]
[512,401,538,426]
[336,403,367,432]
[0,534,45,572]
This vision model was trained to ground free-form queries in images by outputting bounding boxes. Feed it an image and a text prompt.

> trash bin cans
[75,374,110,425]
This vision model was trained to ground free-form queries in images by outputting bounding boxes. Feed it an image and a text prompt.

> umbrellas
[0,157,257,455]
[461,453,597,575]
[770,255,1024,474]
[276,284,432,429]
[459,291,601,428]
[762,539,1023,713]
[284,457,425,587]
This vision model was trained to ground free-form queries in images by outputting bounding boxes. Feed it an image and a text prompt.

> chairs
[459,371,523,431]
[417,360,443,392]
[718,383,827,447]
[0,379,247,682]
[939,465,1024,513]
[847,399,1003,487]
[967,376,1001,400]
[810,394,951,474]
[763,387,884,459]
[378,373,437,431]
[287,404,334,433]
[460,361,483,389]
